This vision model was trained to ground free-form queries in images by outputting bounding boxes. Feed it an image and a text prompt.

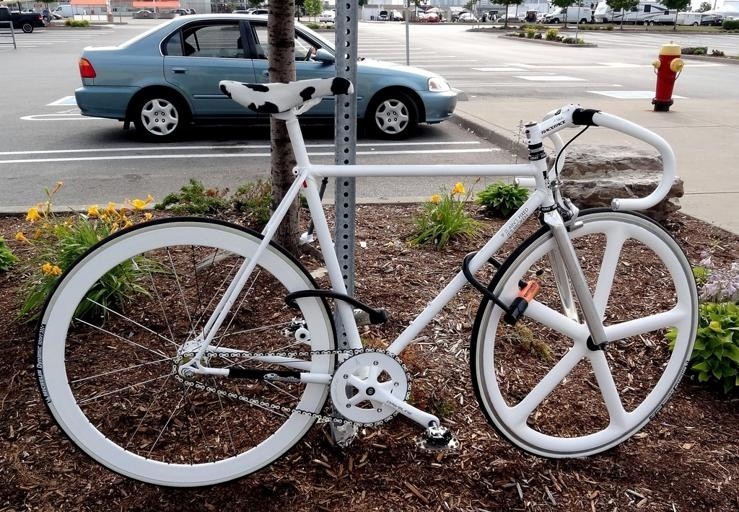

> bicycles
[29,74,703,492]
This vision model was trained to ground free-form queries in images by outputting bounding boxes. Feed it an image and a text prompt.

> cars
[0,6,61,34]
[702,14,722,26]
[388,10,550,23]
[318,10,335,24]
[72,11,461,144]
[132,3,269,18]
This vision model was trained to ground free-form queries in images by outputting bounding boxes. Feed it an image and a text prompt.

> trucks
[546,1,702,27]
[53,5,86,17]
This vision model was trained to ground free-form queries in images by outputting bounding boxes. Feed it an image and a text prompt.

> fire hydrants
[649,39,685,113]
